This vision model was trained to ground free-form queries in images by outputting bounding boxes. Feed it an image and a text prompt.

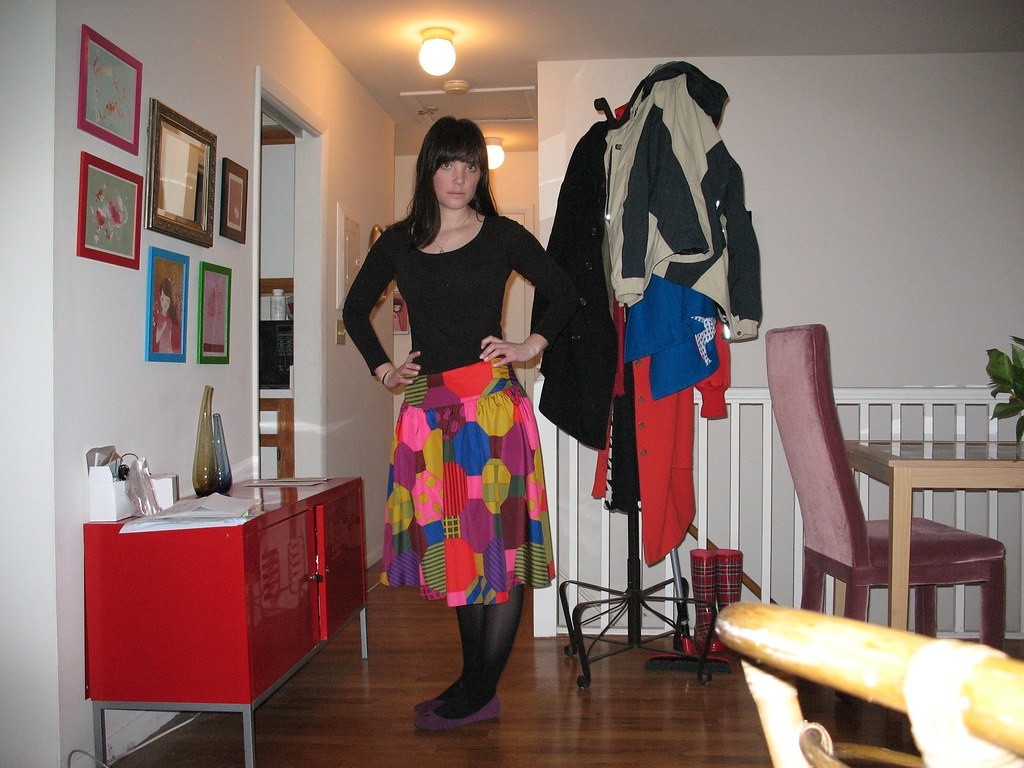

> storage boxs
[84,445,133,521]
[151,473,180,510]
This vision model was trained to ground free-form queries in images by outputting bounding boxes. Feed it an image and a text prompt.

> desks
[832,438,1024,631]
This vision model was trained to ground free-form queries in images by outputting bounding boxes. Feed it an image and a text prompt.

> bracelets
[381,369,392,386]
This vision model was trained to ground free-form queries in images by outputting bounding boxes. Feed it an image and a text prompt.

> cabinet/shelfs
[260,398,295,478]
[82,477,369,768]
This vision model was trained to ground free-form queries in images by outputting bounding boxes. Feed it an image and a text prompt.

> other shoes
[415,692,500,730]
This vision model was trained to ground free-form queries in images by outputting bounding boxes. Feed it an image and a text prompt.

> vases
[212,412,233,494]
[191,384,221,497]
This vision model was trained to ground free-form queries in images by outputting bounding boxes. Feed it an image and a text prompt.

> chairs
[767,323,1008,649]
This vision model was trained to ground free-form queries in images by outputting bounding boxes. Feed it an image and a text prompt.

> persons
[341,116,581,733]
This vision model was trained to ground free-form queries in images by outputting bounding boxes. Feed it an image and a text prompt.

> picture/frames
[220,157,248,246]
[76,24,144,156]
[75,150,144,271]
[144,245,191,363]
[336,201,364,310]
[196,260,233,364]
[143,97,218,248]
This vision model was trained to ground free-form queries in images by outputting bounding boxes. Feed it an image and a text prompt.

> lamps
[419,27,456,75]
[485,137,506,170]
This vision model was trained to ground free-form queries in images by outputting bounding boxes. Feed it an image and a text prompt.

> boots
[689,549,744,653]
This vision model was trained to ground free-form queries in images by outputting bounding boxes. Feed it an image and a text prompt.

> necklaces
[433,227,453,254]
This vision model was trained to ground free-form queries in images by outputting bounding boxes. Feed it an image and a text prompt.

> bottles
[211,413,232,493]
[193,385,218,498]
[270,289,285,320]
